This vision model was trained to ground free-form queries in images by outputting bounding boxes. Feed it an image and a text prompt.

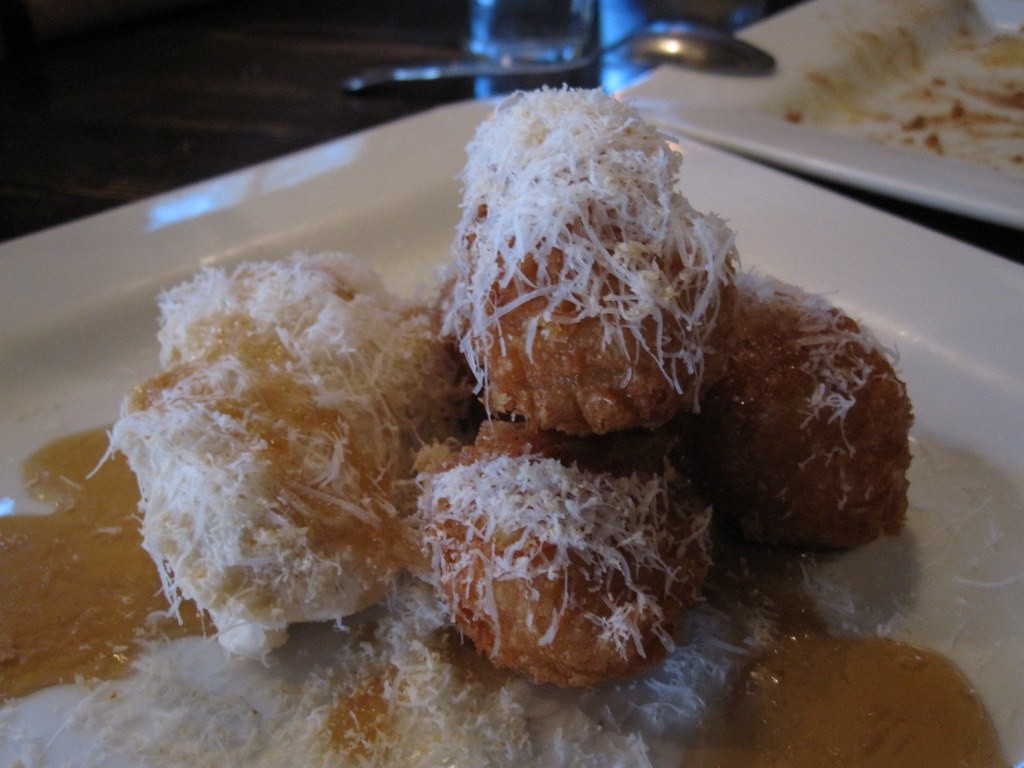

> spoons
[338,19,777,103]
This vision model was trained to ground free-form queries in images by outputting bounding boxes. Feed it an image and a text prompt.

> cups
[470,0,597,96]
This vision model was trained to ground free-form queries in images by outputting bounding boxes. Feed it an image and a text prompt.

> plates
[609,0,1024,232]
[1,100,1024,768]
